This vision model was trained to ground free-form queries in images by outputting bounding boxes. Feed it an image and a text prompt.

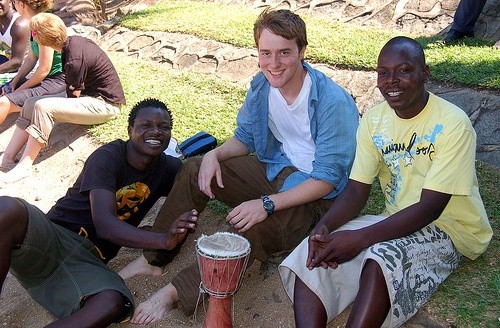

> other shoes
[441,28,474,44]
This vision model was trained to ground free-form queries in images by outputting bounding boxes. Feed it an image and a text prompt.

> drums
[196,231,251,328]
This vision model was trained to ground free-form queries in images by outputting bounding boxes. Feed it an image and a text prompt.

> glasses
[13,1,20,5]
[32,30,38,37]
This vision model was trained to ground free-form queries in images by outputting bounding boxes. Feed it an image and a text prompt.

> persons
[118,10,360,325]
[278,36,493,328]
[0,97,198,328]
[0,0,66,124]
[445,0,487,44]
[0,13,127,184]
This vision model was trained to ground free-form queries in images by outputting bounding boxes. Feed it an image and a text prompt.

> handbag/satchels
[177,132,217,157]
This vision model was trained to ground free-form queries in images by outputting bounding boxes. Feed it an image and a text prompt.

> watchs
[260,194,276,216]
